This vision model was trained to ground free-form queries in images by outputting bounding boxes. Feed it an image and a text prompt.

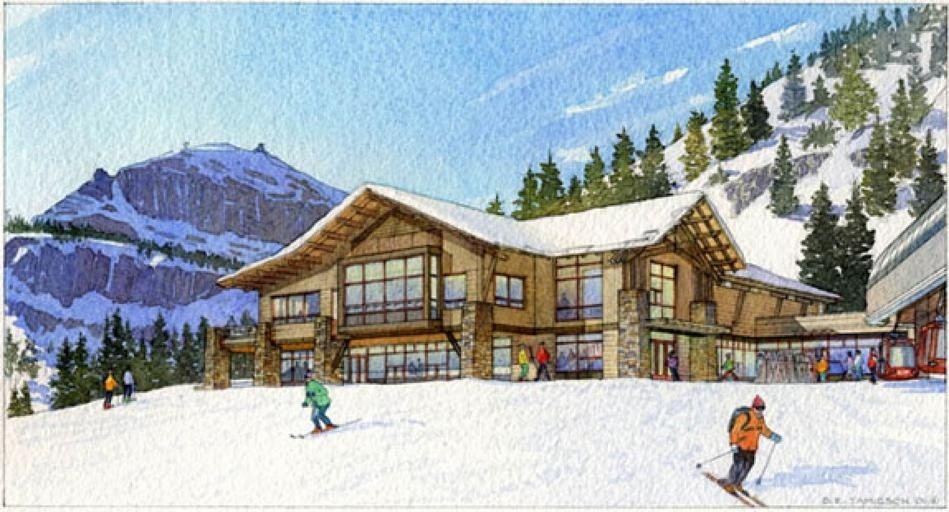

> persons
[534,342,551,380]
[665,351,684,381]
[816,347,880,384]
[715,353,739,381]
[408,360,416,373]
[717,395,783,496]
[103,370,118,408]
[300,370,334,433]
[123,365,134,402]
[518,345,529,381]
[414,358,422,372]
[556,294,603,372]
